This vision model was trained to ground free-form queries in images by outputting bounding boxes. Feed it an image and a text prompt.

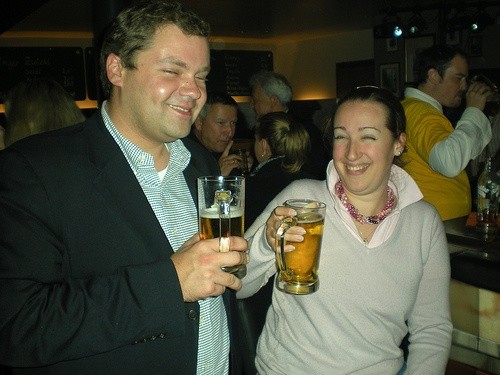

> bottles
[477,155,493,227]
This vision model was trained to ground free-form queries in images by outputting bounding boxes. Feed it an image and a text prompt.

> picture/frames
[379,62,400,100]
[466,35,482,57]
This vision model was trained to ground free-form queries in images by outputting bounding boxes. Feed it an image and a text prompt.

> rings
[233,159,235,164]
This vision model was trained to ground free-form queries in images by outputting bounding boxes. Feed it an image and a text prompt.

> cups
[198,175,247,279]
[463,72,499,102]
[228,148,249,175]
[275,199,326,295]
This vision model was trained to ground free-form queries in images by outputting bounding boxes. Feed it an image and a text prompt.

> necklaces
[334,181,395,224]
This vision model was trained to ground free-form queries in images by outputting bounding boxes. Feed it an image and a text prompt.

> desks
[442,214,500,375]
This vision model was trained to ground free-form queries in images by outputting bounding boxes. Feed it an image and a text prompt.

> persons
[0,0,250,375]
[189,90,242,176]
[247,67,317,172]
[311,108,337,180]
[392,42,493,224]
[4,75,87,146]
[235,86,452,375]
[242,111,316,234]
